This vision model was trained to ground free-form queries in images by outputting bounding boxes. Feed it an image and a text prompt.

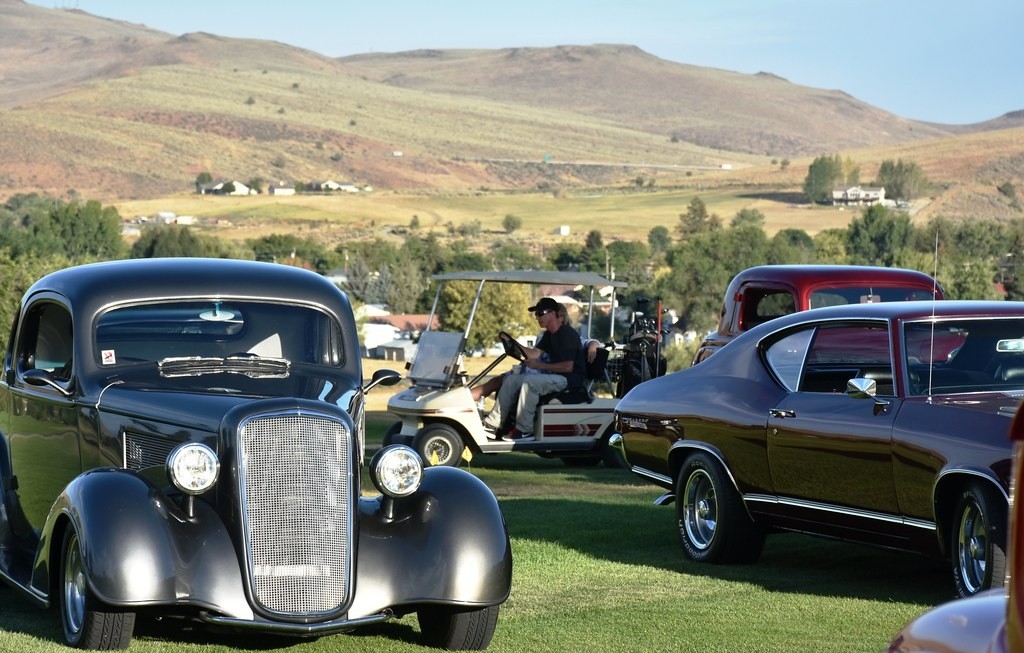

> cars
[607,299,1024,604]
[689,265,970,370]
[0,257,512,651]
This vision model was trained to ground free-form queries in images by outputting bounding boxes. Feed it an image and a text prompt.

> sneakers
[502,427,535,441]
[482,420,497,439]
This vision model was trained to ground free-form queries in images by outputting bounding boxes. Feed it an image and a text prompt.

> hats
[528,298,559,312]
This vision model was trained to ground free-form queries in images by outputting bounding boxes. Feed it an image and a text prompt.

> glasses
[535,309,553,317]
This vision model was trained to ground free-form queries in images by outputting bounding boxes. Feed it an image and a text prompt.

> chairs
[908,364,999,395]
[210,351,289,400]
[542,346,608,405]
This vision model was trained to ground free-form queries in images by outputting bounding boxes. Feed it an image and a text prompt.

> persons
[471,297,601,441]
